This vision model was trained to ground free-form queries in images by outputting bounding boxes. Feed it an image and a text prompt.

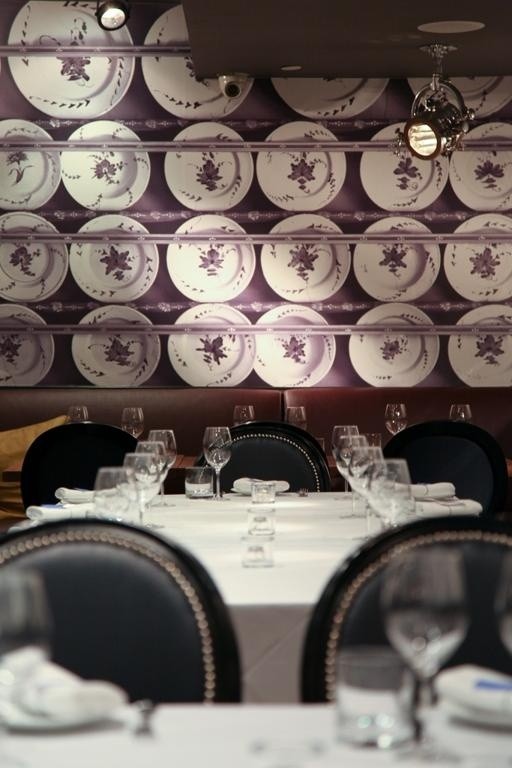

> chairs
[20,420,166,518]
[298,513,512,704]
[190,419,333,493]
[0,516,243,703]
[375,419,508,513]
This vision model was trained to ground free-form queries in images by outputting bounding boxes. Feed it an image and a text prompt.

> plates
[447,302,512,388]
[60,117,151,213]
[165,214,258,305]
[0,303,55,385]
[347,303,441,388]
[255,121,348,214]
[449,121,512,213]
[247,305,337,388]
[162,121,255,214]
[168,303,257,390]
[267,78,392,123]
[364,120,450,212]
[73,306,160,390]
[443,214,512,302]
[352,216,442,304]
[403,78,512,121]
[6,1,137,120]
[68,214,162,306]
[1,212,68,302]
[257,214,352,306]
[1,119,62,212]
[137,1,264,119]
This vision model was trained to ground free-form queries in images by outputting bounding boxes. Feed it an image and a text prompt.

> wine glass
[89,426,174,530]
[203,427,234,502]
[330,425,417,542]
[375,546,475,762]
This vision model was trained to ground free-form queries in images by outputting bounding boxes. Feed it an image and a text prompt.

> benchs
[282,386,512,490]
[0,387,283,471]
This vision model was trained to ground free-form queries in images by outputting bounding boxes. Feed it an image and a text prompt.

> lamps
[395,43,476,160]
[95,0,129,31]
[218,75,242,100]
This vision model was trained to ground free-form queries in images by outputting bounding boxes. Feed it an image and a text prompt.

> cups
[286,405,306,435]
[336,646,413,747]
[385,404,407,435]
[182,462,215,500]
[70,406,89,424]
[121,407,145,440]
[232,405,256,427]
[445,403,472,423]
[239,478,277,578]
